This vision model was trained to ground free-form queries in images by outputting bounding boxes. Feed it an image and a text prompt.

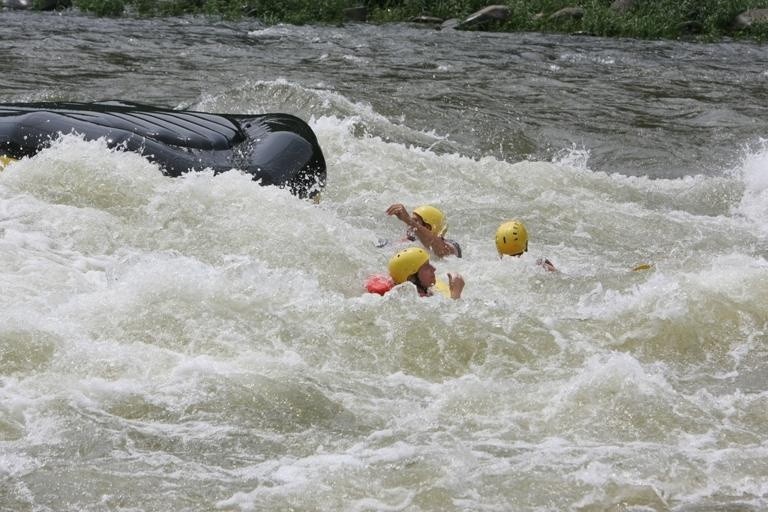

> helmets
[412,204,446,238]
[494,219,530,256]
[387,246,431,286]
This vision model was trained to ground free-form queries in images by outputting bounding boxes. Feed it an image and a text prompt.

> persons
[495,221,561,275]
[370,246,466,303]
[384,204,462,260]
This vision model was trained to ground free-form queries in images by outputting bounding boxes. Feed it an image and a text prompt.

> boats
[0,97,331,211]
